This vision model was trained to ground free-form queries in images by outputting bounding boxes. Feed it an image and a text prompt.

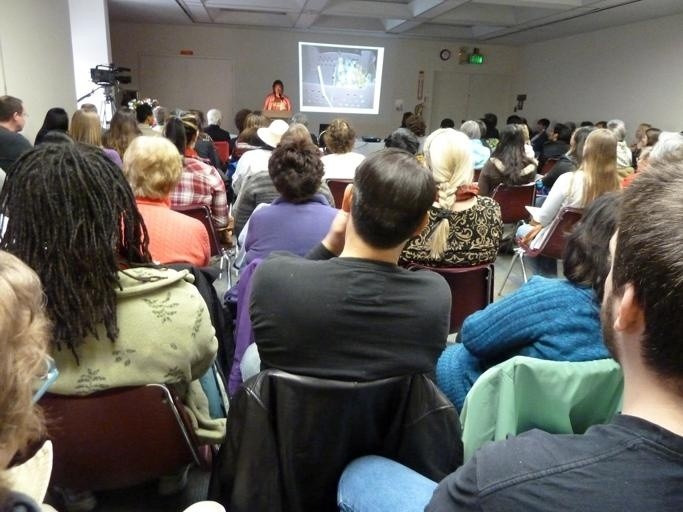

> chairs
[539,156,560,176]
[493,357,624,444]
[132,259,231,418]
[498,207,584,297]
[32,382,204,512]
[175,202,222,257]
[402,262,493,342]
[491,181,537,224]
[213,141,230,164]
[324,179,355,213]
[209,368,412,512]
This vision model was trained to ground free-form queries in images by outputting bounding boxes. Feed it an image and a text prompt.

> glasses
[32,354,60,405]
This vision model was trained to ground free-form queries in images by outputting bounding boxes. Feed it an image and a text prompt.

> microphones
[279,93,284,99]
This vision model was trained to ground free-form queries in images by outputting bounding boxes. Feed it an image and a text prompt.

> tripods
[99,86,117,129]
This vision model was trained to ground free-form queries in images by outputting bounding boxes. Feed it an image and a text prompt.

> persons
[261,78,291,111]
[0,93,683,512]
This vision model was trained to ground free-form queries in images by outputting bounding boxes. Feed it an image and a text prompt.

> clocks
[440,49,450,61]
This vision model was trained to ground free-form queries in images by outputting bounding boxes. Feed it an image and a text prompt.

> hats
[206,109,222,126]
[256,119,290,149]
